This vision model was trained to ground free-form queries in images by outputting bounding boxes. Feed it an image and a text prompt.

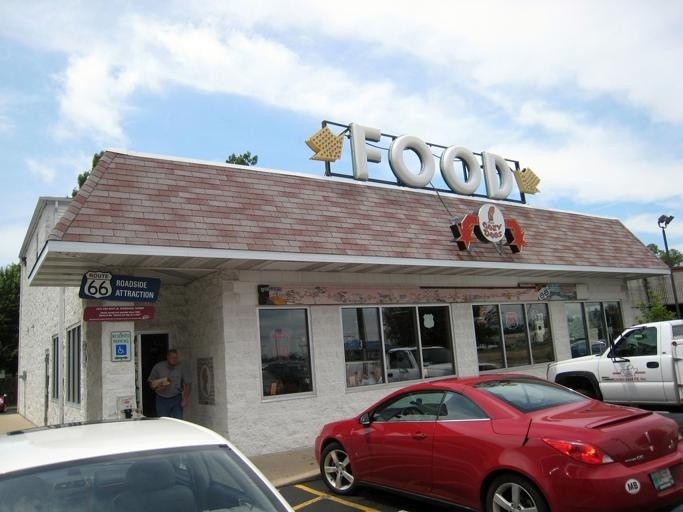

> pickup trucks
[546,320,683,407]
[346,346,499,382]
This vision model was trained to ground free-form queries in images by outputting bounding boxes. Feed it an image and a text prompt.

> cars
[262,362,311,395]
[546,336,605,359]
[0,414,293,511]
[314,372,682,511]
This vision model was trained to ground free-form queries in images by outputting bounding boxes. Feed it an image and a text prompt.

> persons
[362,372,367,379]
[368,372,376,384]
[147,349,193,419]
[349,372,360,385]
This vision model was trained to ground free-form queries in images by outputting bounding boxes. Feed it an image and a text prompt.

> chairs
[111,459,210,512]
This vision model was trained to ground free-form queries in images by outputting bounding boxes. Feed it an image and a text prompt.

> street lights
[657,215,680,320]
